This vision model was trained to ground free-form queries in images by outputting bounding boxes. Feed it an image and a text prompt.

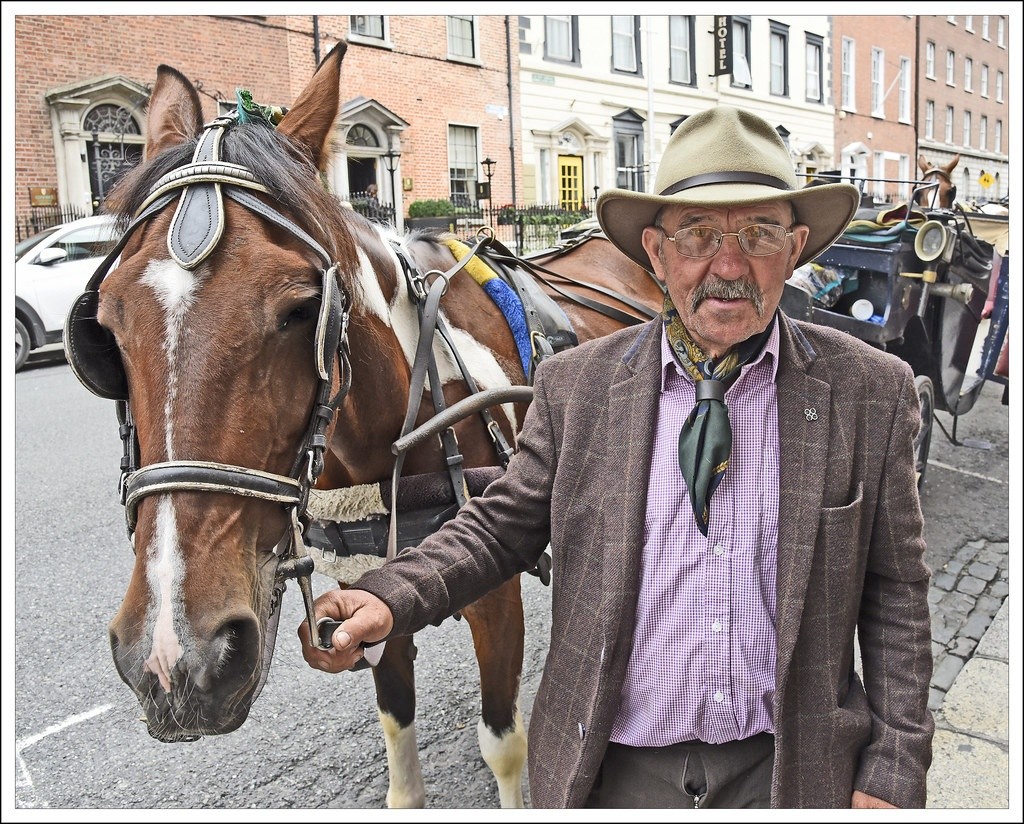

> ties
[661,289,776,538]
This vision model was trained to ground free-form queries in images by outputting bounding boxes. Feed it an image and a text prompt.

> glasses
[659,224,793,259]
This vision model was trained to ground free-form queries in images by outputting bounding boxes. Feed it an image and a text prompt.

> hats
[596,108,862,274]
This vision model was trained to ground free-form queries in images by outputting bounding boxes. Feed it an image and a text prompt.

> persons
[366,183,379,206]
[299,108,934,810]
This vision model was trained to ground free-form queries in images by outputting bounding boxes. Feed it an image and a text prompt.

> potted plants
[404,200,458,230]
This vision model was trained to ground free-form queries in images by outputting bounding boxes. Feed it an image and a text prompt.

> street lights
[377,149,401,230]
[477,157,498,239]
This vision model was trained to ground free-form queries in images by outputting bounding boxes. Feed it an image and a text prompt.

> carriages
[67,38,1009,743]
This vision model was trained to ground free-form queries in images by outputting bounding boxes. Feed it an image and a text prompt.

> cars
[14,212,136,372]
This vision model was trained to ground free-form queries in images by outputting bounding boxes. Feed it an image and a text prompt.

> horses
[914,153,961,209]
[96,40,664,809]
